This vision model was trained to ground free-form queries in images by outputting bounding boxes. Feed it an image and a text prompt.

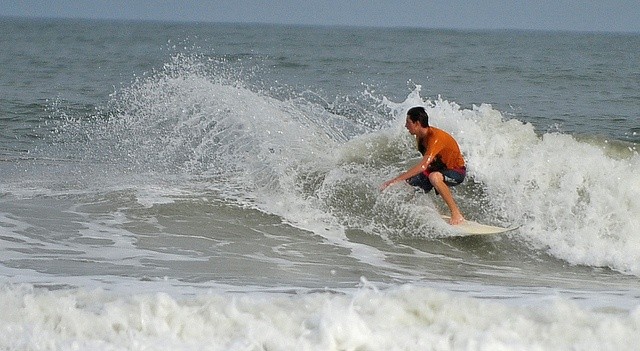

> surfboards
[437,213,520,235]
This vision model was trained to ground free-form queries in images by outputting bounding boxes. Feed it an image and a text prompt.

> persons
[383,106,466,225]
